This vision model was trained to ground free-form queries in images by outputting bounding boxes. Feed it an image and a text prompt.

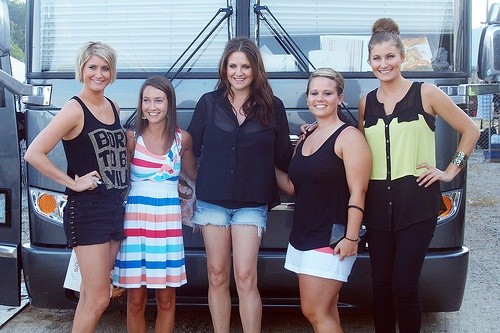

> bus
[0,0,499,314]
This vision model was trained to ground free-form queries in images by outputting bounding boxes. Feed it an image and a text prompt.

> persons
[186,36,305,333]
[298,18,481,333]
[275,67,372,333]
[112,75,198,333]
[24,41,128,333]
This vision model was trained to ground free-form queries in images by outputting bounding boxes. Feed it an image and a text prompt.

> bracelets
[451,151,469,169]
[347,203,365,216]
[345,236,359,241]
